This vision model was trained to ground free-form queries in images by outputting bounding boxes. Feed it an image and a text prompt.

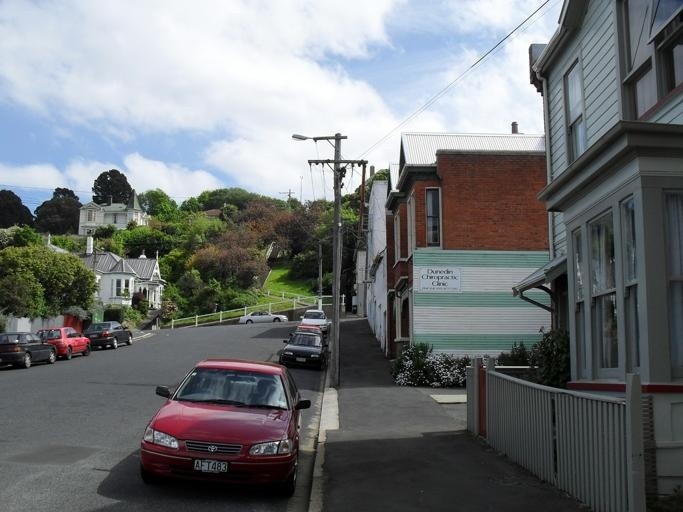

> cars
[238,311,289,324]
[300,310,331,332]
[83,321,133,349]
[0,332,57,369]
[278,330,328,371]
[138,357,311,498]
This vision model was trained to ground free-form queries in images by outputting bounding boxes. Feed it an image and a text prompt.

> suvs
[37,326,91,360]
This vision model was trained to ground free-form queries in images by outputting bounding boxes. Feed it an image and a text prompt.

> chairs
[248,379,278,405]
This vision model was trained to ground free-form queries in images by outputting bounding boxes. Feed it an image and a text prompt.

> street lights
[291,132,348,389]
